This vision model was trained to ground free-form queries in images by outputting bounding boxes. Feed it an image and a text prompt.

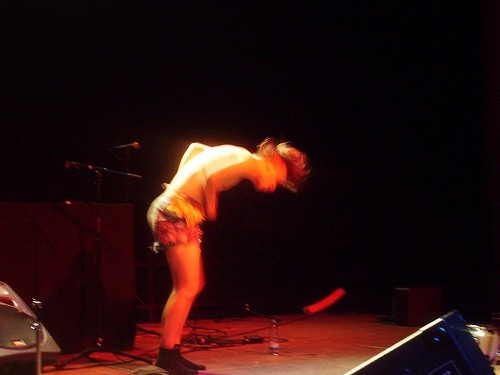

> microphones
[64,159,83,170]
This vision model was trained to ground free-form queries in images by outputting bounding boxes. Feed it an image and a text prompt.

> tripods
[53,171,154,371]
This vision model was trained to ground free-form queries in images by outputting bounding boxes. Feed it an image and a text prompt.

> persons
[145,136,311,375]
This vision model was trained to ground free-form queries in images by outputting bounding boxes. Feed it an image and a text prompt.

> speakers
[0,281,61,369]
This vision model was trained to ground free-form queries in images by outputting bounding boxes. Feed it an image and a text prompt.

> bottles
[270,320,280,357]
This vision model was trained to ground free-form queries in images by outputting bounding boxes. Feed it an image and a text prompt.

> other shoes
[155,347,199,375]
[175,344,206,370]
